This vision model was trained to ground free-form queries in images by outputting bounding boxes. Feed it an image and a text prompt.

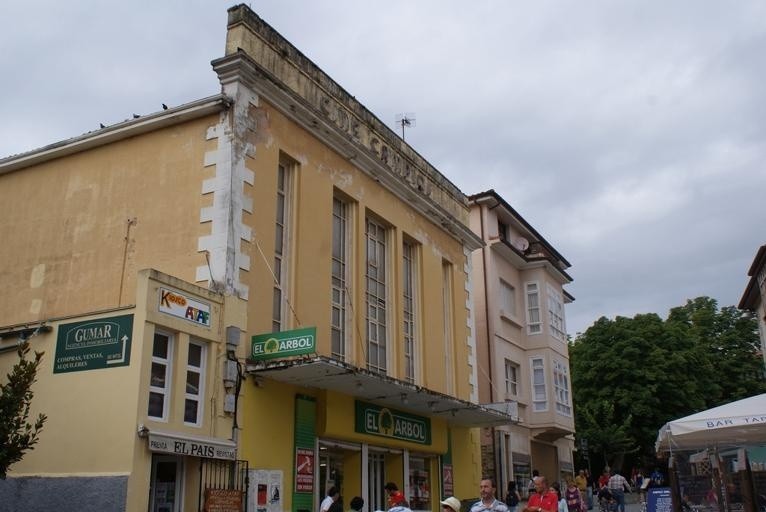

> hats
[439,496,461,512]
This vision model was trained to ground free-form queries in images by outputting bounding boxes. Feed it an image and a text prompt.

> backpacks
[505,489,518,507]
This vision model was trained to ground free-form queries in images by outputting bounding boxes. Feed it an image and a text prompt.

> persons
[384,482,411,511]
[631,468,662,503]
[471,474,508,511]
[523,464,632,511]
[321,486,344,511]
[441,497,461,511]
[350,495,364,512]
